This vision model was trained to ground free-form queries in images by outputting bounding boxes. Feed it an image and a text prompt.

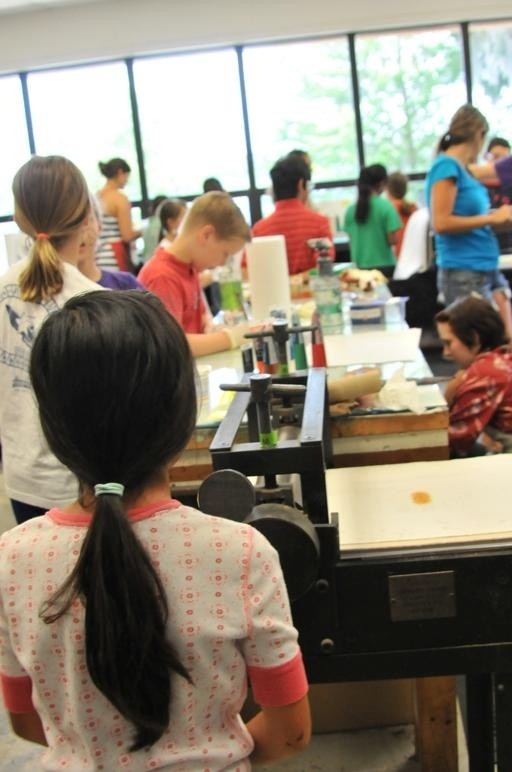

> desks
[166,284,512,770]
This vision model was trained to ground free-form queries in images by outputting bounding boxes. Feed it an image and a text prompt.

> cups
[194,363,213,412]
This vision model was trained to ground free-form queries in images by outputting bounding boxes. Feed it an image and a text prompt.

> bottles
[313,257,344,335]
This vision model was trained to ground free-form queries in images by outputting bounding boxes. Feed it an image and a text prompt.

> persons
[425,103,509,339]
[434,298,511,454]
[95,159,144,271]
[134,191,258,361]
[3,155,122,524]
[470,139,512,281]
[145,178,245,315]
[73,193,144,292]
[244,148,443,326]
[1,288,312,770]
